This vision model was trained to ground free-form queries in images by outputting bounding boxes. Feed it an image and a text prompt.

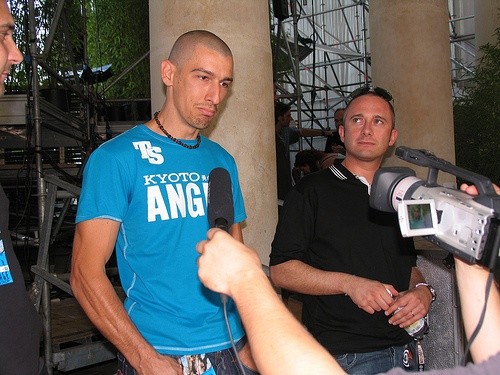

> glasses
[349,85,394,101]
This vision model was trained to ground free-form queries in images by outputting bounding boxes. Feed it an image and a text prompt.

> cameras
[369,145,500,291]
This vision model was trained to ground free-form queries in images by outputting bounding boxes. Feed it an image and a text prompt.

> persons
[69,30,255,375]
[0,0,44,375]
[195,182,500,375]
[409,207,426,229]
[268,91,433,375]
[274,102,346,212]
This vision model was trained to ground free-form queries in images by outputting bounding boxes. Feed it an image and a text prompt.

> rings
[411,311,415,317]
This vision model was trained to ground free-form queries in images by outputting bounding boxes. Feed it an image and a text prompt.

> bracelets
[345,275,355,296]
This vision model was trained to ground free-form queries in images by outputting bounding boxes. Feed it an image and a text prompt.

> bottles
[386,289,431,341]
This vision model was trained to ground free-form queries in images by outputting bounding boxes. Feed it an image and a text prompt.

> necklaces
[152,110,201,149]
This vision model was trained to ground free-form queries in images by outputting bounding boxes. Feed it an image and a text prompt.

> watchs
[415,282,437,302]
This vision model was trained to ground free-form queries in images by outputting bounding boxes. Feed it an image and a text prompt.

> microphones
[208,167,234,304]
